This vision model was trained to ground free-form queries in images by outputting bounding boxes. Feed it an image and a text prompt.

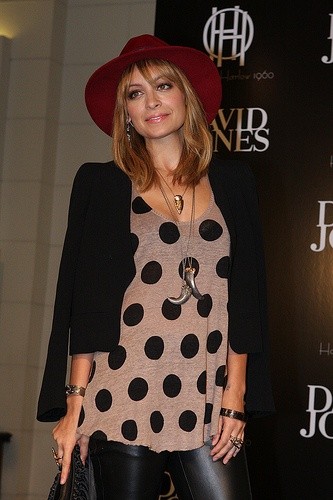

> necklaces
[151,167,202,307]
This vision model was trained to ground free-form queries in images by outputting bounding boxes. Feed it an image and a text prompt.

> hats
[85,34,222,137]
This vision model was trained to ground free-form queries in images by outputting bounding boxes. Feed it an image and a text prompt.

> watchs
[65,383,86,397]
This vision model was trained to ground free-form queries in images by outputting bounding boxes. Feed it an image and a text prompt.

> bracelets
[220,407,246,421]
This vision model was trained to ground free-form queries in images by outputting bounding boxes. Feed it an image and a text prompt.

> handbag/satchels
[48,443,101,500]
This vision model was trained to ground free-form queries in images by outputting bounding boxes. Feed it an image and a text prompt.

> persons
[34,33,247,500]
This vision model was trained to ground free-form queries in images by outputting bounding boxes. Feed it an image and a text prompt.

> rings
[230,435,244,449]
[52,447,63,466]
